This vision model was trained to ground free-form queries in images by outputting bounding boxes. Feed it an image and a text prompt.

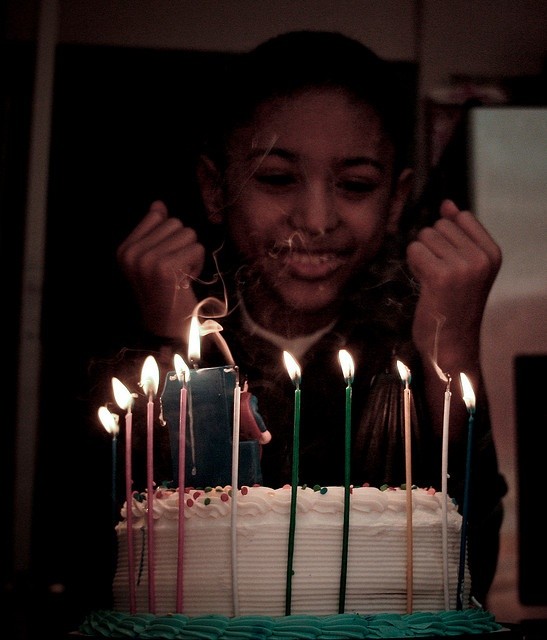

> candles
[433,358,452,612]
[98,406,120,503]
[230,366,241,618]
[111,375,138,616]
[283,350,302,616]
[160,314,262,486]
[141,355,159,614]
[397,361,413,616]
[174,353,190,614]
[456,371,477,610]
[339,348,356,614]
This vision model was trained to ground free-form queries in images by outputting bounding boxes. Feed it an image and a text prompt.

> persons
[91,30,504,613]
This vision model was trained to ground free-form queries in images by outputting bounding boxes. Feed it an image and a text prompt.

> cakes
[78,484,503,640]
